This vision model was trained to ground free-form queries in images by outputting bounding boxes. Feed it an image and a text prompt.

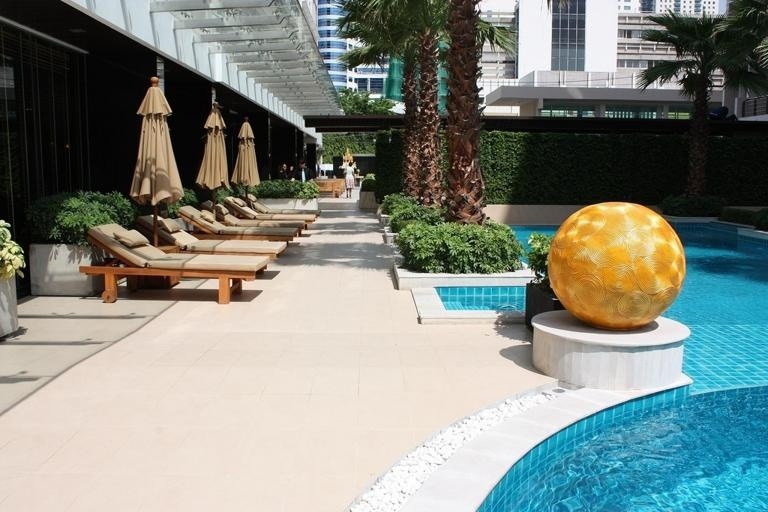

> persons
[344,162,355,199]
[290,158,315,183]
[279,163,289,179]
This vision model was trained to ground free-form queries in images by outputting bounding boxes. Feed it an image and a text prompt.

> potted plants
[358,178,378,208]
[523,229,564,331]
[0,218,28,344]
[252,178,320,210]
[26,185,139,299]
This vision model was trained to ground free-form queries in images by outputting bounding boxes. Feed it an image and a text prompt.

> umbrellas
[130,74,185,248]
[194,100,232,218]
[229,114,262,205]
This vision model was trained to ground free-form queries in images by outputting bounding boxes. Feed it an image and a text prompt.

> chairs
[316,178,346,198]
[76,194,322,306]
